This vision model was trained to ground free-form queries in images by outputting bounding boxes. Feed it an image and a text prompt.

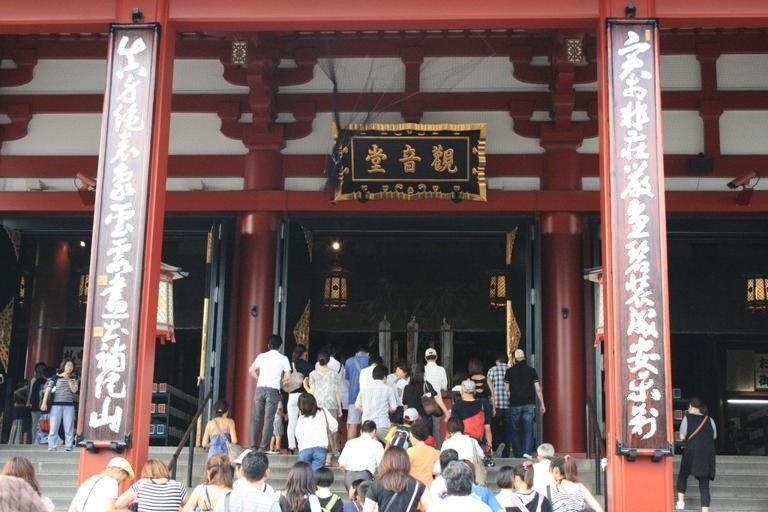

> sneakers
[675,501,684,510]
[522,454,531,459]
[493,443,504,459]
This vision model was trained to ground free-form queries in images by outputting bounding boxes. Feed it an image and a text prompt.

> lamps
[744,238,768,313]
[487,238,510,312]
[319,240,352,312]
[78,236,89,304]
[19,235,26,304]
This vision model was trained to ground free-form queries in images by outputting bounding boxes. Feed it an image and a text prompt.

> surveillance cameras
[75,172,98,192]
[726,168,757,195]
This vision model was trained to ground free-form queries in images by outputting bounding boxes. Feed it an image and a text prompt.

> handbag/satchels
[473,457,486,484]
[227,444,242,469]
[420,381,441,417]
[327,429,342,455]
[281,362,303,392]
[39,420,49,432]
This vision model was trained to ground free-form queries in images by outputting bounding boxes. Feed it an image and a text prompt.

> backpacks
[389,426,411,450]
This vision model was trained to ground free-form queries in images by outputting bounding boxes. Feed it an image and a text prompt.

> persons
[675,397,717,512]
[0,456,55,512]
[231,336,600,511]
[6,358,79,452]
[201,399,238,458]
[67,457,134,512]
[113,458,190,512]
[179,453,235,511]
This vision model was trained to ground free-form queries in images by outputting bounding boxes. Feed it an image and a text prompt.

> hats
[512,349,525,361]
[402,408,418,422]
[461,381,475,393]
[424,348,436,357]
[107,457,134,481]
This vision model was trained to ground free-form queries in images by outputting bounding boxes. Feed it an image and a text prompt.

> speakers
[688,156,714,173]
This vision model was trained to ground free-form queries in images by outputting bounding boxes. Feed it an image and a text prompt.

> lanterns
[741,271,768,311]
[487,269,508,312]
[321,263,350,312]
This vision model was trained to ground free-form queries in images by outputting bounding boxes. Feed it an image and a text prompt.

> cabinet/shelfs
[673,388,690,454]
[150,383,198,445]
